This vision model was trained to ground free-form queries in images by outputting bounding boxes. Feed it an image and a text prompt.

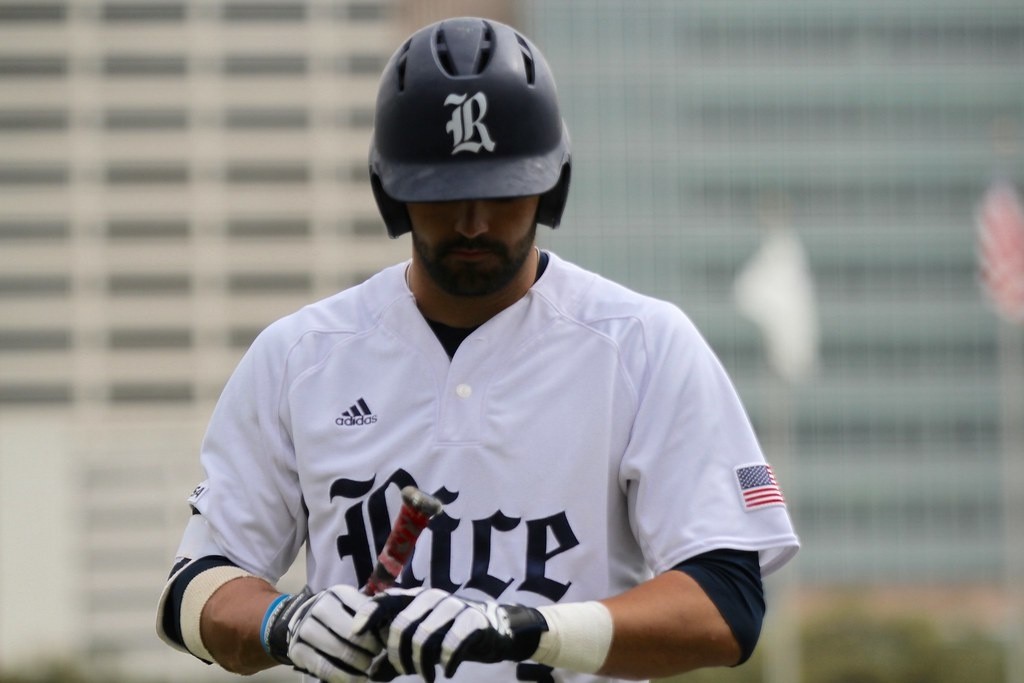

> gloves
[351,587,539,683]
[268,583,391,683]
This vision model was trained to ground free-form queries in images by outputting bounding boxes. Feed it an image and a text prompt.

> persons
[156,18,800,683]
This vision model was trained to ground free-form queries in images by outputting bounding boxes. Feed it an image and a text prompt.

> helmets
[366,16,573,238]
